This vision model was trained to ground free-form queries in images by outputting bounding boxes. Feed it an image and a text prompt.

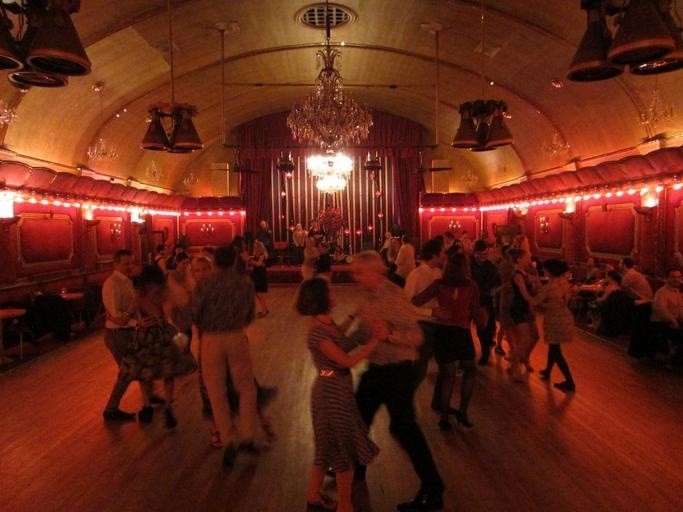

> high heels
[431,402,473,432]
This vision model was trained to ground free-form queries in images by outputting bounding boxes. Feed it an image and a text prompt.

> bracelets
[347,312,356,321]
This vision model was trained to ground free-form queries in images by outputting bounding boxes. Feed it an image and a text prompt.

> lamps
[140,0,204,156]
[537,78,571,157]
[183,153,201,187]
[306,152,353,179]
[450,2,513,153]
[145,152,164,180]
[636,74,676,126]
[459,152,479,187]
[284,0,374,151]
[315,175,346,195]
[566,0,682,84]
[0,0,94,89]
[87,82,120,162]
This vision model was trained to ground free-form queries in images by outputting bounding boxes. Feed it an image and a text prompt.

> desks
[0,281,108,368]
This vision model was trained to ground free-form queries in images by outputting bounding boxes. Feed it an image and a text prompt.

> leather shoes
[103,396,177,428]
[223,440,259,472]
[479,347,505,363]
[396,492,444,511]
[555,381,575,391]
[306,493,336,512]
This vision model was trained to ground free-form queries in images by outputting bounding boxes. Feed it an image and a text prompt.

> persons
[411,252,490,433]
[291,277,388,512]
[153,242,216,344]
[576,256,681,367]
[102,250,145,420]
[325,251,445,511]
[103,266,198,430]
[444,229,576,393]
[193,245,258,477]
[389,235,458,415]
[381,228,415,286]
[233,220,272,294]
[292,223,343,281]
[188,252,279,451]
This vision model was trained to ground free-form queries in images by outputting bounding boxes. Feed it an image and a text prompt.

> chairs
[625,299,681,374]
[583,287,634,340]
